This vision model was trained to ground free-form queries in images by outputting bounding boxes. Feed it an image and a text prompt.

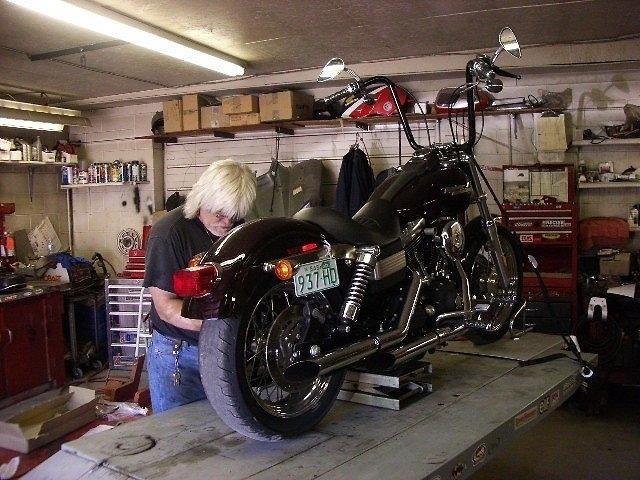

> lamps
[0,99,95,135]
[1,0,248,79]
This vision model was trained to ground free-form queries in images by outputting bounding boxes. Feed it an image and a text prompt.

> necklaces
[202,225,222,246]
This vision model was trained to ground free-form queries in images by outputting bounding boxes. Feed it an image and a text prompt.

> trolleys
[65,295,102,380]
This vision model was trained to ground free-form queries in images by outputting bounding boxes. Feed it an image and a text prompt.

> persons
[140,157,259,414]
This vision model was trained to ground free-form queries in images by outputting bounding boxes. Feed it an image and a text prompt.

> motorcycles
[171,28,523,444]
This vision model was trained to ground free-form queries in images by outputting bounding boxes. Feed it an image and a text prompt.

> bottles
[35,136,42,162]
[60,160,147,184]
[46,239,54,255]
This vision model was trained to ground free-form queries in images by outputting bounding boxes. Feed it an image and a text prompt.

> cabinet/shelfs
[1,290,65,417]
[500,161,580,338]
[573,133,639,233]
[74,280,120,376]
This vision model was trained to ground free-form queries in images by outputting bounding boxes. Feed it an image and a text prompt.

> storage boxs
[600,252,630,276]
[0,385,98,455]
[162,90,315,135]
